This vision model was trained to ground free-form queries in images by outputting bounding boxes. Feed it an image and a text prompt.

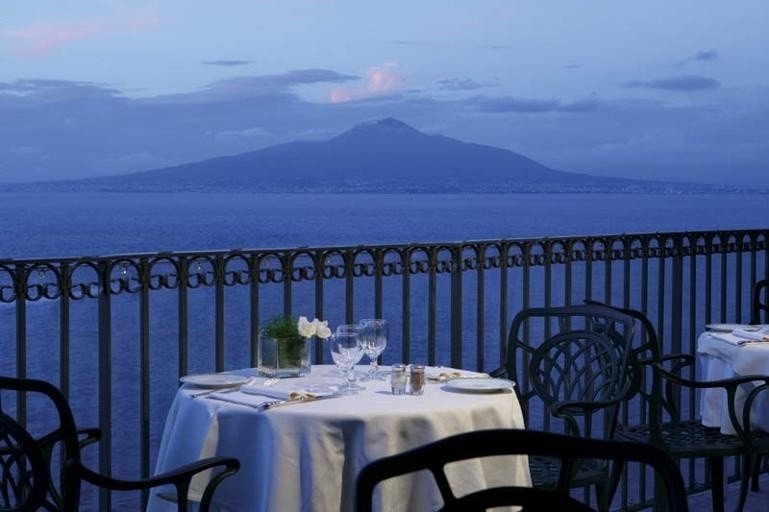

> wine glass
[330,318,387,393]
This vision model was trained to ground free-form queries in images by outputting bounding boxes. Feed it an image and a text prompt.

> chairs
[480,304,636,511]
[581,297,768,511]
[354,425,690,511]
[0,376,240,512]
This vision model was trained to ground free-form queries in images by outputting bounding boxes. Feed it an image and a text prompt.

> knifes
[191,389,220,398]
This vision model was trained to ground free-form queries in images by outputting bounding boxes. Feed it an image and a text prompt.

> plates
[705,323,760,332]
[445,378,516,393]
[179,373,252,389]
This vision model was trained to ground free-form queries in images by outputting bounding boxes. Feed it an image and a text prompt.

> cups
[391,364,425,395]
